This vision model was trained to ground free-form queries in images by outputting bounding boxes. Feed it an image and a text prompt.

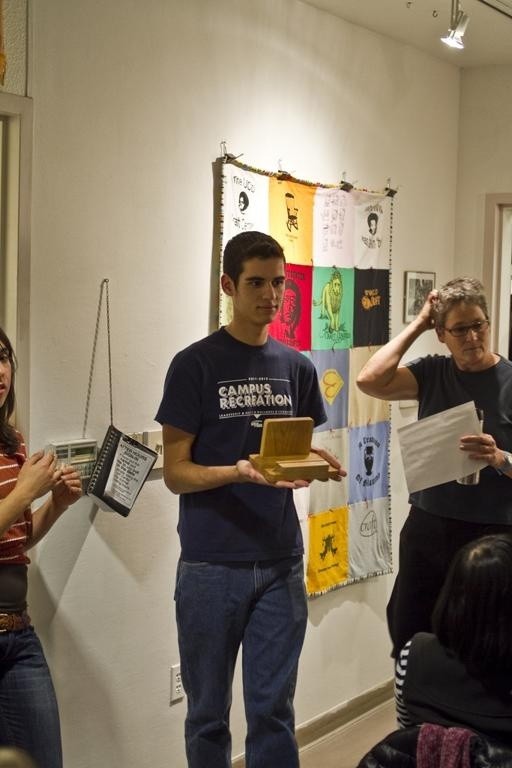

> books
[86,425,158,517]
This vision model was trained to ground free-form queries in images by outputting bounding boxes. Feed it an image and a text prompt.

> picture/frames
[404,270,439,325]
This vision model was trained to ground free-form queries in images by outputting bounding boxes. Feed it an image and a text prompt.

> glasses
[441,320,489,337]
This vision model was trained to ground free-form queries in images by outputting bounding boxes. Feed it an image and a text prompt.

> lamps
[441,0,470,50]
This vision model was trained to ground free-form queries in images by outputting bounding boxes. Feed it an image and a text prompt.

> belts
[0,611,31,633]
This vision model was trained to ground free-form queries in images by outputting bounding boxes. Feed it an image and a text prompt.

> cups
[456,406,485,485]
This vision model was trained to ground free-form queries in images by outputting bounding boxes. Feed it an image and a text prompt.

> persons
[155,231,346,768]
[356,277,512,658]
[395,535,512,730]
[0,327,82,768]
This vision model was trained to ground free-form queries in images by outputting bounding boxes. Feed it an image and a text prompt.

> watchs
[496,451,512,476]
